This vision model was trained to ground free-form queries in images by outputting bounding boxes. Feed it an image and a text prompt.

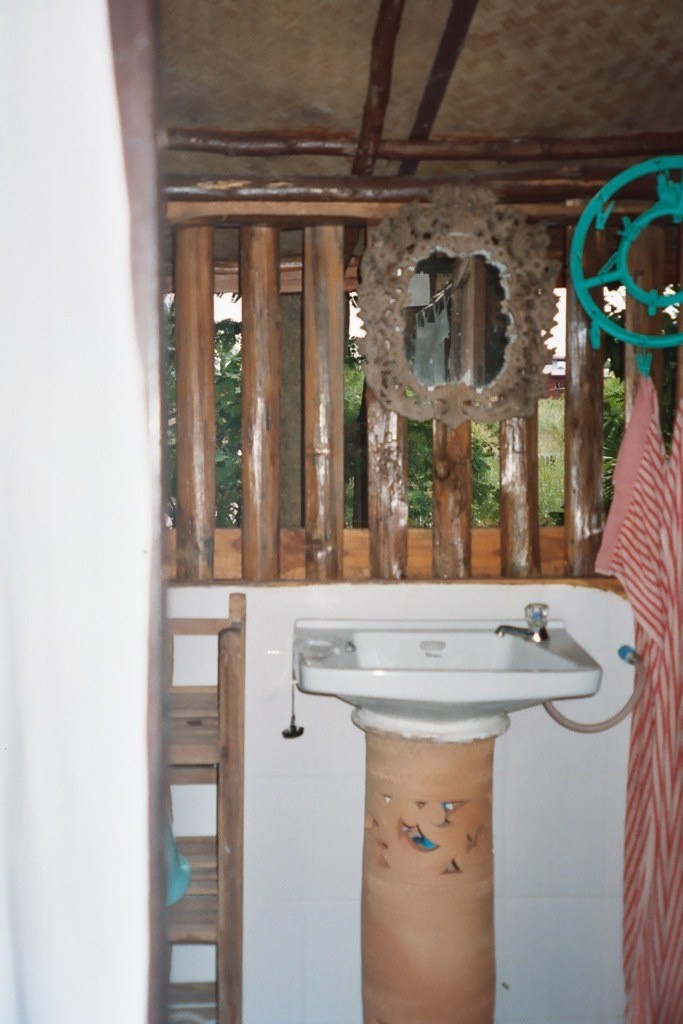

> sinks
[294,616,597,715]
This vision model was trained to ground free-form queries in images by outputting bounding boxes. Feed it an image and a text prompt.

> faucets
[496,605,550,642]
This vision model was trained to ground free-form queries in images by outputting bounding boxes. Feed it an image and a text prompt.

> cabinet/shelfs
[147,590,246,1024]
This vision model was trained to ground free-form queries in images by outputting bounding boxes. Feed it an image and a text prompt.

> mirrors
[355,183,563,428]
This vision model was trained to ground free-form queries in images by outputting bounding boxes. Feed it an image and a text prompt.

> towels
[597,371,681,1023]
